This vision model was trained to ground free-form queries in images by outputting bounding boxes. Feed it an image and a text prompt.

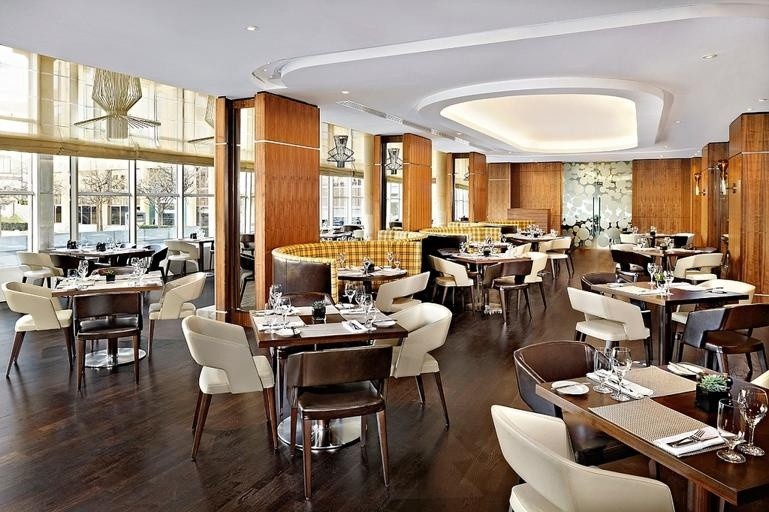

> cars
[322,215,363,233]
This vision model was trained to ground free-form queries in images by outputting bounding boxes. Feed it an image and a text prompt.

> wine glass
[263,283,396,338]
[717,386,768,465]
[459,224,557,258]
[593,346,633,401]
[645,262,675,295]
[337,252,402,275]
[631,225,674,250]
[76,257,149,285]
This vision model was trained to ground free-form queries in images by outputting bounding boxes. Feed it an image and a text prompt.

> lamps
[718,159,736,196]
[695,173,705,196]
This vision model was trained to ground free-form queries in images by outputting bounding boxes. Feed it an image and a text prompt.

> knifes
[672,434,718,449]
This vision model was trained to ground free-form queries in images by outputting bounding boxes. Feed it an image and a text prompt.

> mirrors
[232,108,256,313]
[382,142,403,229]
[454,158,469,221]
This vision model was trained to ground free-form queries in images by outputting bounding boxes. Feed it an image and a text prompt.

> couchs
[478,220,535,231]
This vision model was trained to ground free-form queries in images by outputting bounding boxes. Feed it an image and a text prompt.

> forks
[667,429,705,445]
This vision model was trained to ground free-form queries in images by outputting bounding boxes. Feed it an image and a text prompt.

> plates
[668,361,702,376]
[550,379,590,397]
